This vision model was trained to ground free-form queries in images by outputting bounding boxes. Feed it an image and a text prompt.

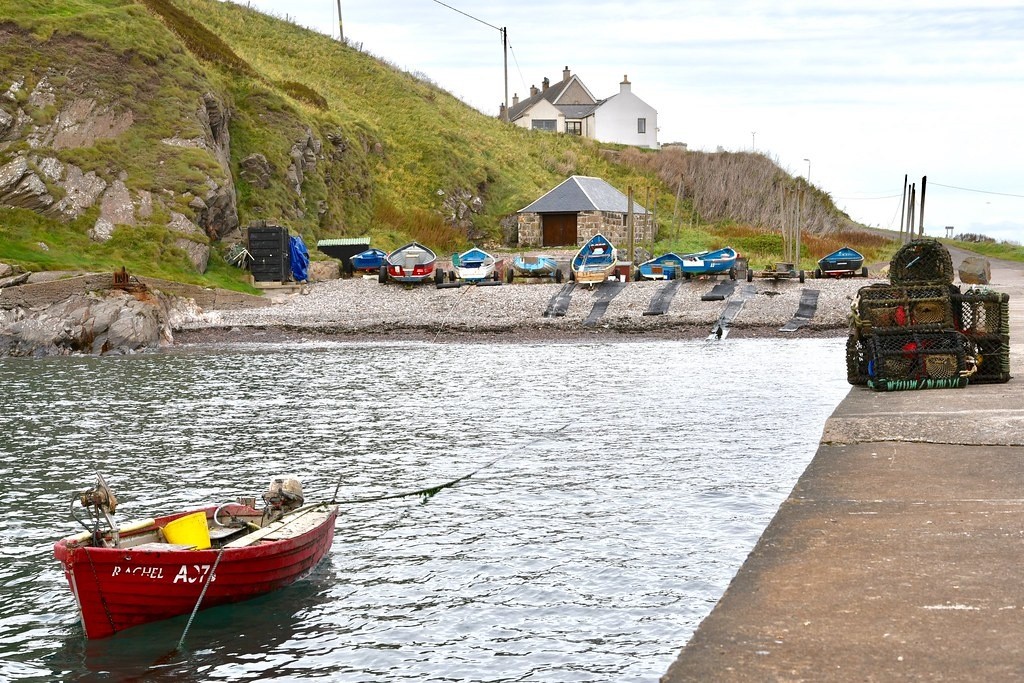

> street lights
[803,159,811,182]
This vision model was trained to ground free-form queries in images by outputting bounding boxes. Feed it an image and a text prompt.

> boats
[817,246,864,276]
[385,241,437,283]
[638,251,684,280]
[682,245,738,277]
[452,246,495,281]
[512,255,558,276]
[349,247,387,272]
[53,468,340,641]
[571,232,617,286]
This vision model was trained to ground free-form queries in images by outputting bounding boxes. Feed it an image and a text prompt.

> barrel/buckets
[161,512,211,550]
